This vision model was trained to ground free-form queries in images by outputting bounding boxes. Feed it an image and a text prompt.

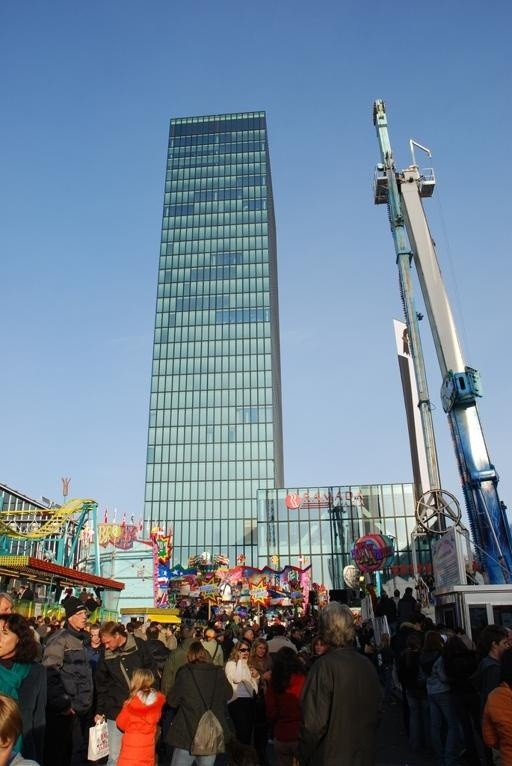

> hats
[63,596,87,619]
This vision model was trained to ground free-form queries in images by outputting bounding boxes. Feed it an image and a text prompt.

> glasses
[238,647,251,652]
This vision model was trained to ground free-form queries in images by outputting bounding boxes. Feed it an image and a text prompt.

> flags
[104,506,142,535]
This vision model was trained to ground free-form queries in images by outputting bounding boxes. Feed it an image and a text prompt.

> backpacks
[189,709,225,755]
[460,668,484,732]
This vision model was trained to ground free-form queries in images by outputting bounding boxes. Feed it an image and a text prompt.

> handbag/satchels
[87,716,110,762]
